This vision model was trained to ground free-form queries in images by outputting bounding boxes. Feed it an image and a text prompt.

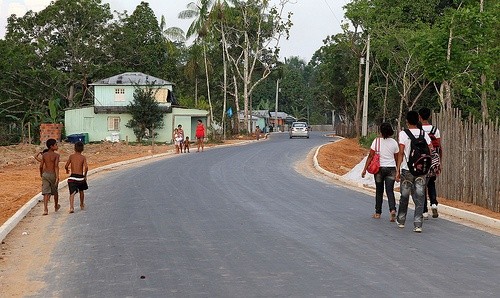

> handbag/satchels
[365,137,381,175]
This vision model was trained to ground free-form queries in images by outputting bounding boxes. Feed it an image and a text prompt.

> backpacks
[427,145,441,177]
[429,125,442,162]
[403,129,432,177]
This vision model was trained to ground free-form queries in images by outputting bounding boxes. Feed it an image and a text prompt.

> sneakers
[412,226,422,232]
[370,213,381,219]
[422,215,428,220]
[395,218,406,228]
[389,210,396,223]
[431,206,439,218]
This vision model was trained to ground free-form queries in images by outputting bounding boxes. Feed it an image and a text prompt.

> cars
[289,121,311,139]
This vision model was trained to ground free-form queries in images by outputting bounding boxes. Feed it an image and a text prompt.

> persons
[64,142,88,213]
[174,119,205,155]
[33,138,60,215]
[361,122,400,221]
[394,107,442,233]
